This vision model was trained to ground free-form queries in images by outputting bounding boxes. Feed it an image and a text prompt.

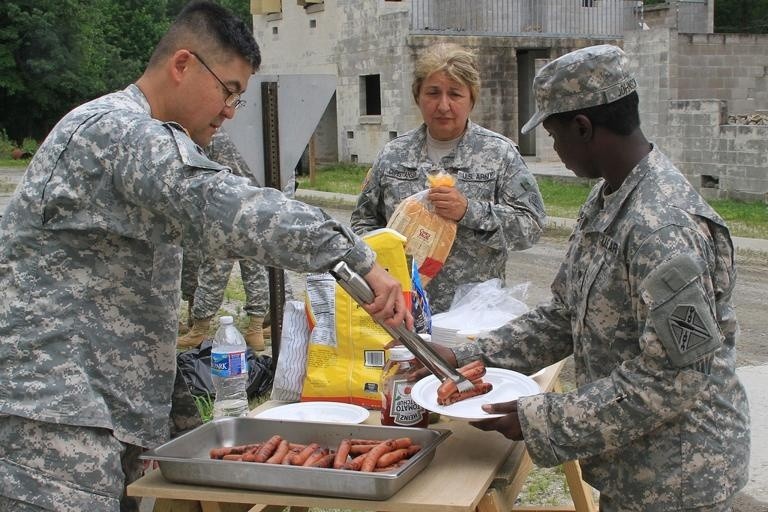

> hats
[520,44,638,135]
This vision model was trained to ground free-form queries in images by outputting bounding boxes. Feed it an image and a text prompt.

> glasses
[188,52,247,111]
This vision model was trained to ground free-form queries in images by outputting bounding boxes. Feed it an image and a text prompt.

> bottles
[377,332,443,429]
[210,315,251,422]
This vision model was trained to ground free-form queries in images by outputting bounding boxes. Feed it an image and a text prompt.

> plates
[427,310,522,348]
[411,364,546,422]
[248,398,371,426]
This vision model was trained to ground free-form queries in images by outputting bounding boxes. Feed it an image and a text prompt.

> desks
[126,354,598,510]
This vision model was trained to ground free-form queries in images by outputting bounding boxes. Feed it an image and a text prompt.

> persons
[177,127,269,352]
[351,44,548,319]
[1,0,416,512]
[381,46,752,511]
[180,238,201,332]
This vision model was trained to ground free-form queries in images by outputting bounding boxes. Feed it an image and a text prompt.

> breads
[386,198,457,289]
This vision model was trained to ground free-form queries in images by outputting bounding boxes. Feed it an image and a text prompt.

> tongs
[330,259,478,399]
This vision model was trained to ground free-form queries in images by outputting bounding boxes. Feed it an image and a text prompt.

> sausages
[435,359,493,405]
[211,434,420,472]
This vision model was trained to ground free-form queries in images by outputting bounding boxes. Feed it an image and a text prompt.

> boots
[177,299,271,351]
